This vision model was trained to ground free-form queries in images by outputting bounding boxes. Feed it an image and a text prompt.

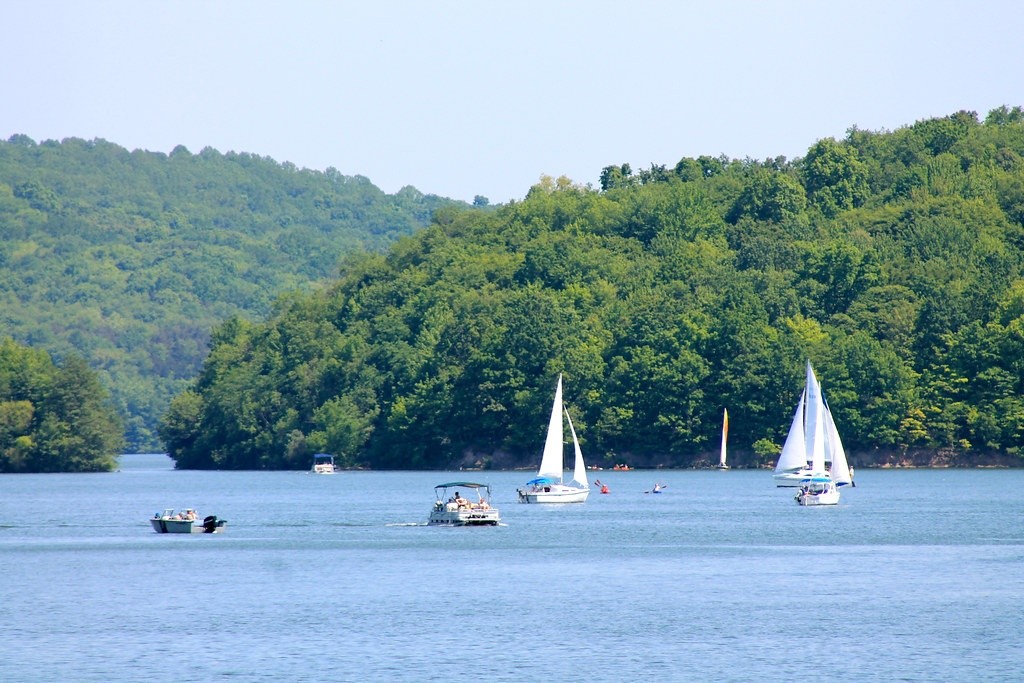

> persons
[655,484,660,490]
[175,510,184,520]
[533,483,539,492]
[603,484,607,489]
[455,492,460,499]
[186,511,195,520]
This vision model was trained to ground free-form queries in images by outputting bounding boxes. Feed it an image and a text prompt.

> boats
[309,453,337,474]
[426,481,503,527]
[795,478,841,506]
[149,508,227,534]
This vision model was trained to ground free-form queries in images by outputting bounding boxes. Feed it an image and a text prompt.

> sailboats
[715,406,731,472]
[771,358,854,489]
[514,371,592,504]
